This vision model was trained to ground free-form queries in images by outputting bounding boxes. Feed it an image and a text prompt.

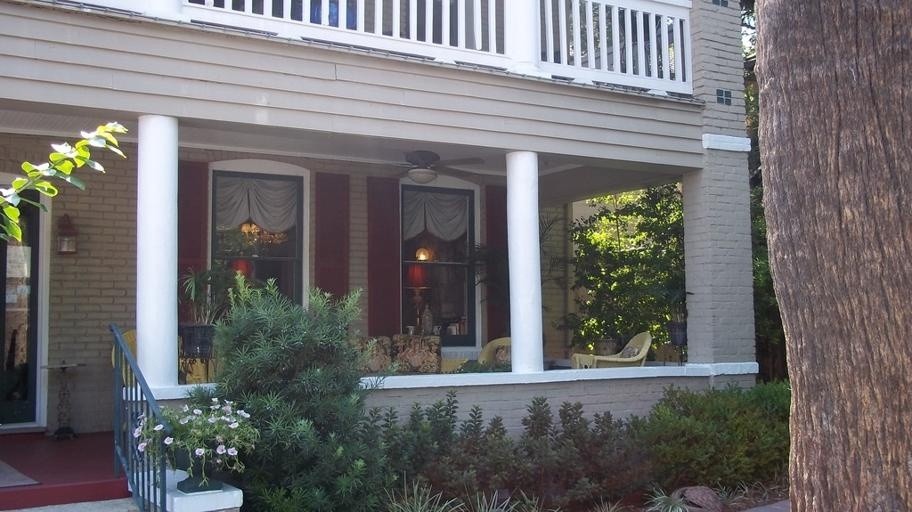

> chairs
[565,331,651,367]
[477,336,511,365]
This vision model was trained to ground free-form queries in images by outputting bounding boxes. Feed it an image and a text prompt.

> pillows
[619,343,638,358]
[494,343,512,364]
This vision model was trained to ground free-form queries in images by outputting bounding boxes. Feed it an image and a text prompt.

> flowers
[133,397,260,492]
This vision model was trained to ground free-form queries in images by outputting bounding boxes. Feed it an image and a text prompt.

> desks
[42,360,88,440]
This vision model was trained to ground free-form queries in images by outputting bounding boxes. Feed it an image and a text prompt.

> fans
[339,151,484,188]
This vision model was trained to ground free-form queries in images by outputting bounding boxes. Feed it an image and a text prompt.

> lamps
[58,215,77,256]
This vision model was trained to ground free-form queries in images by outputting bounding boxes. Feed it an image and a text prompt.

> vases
[667,323,688,346]
[177,452,224,492]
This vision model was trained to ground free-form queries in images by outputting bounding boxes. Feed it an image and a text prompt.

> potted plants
[177,268,230,357]
